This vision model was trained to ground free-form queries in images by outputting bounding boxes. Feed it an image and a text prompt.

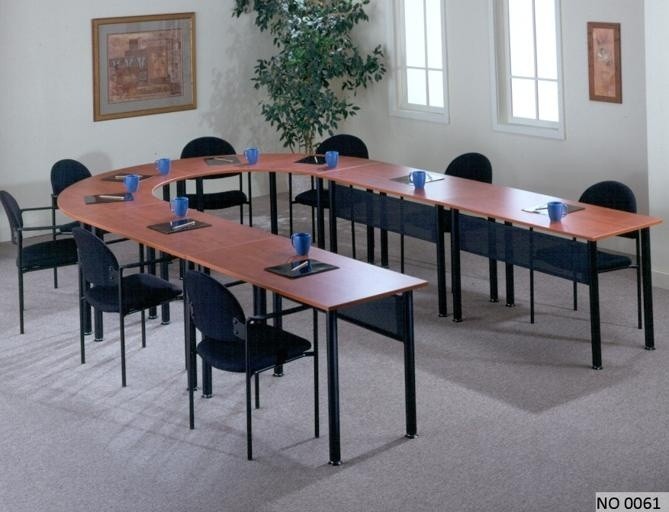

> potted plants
[230,0,390,203]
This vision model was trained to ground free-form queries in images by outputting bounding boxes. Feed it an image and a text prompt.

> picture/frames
[587,22,622,104]
[91,11,196,122]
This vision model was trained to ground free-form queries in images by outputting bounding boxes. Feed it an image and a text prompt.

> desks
[79,202,276,372]
[186,237,429,465]
[57,152,383,336]
[325,162,499,317]
[443,187,663,370]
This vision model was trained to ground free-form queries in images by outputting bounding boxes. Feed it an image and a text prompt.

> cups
[244,148,258,164]
[291,232,311,256]
[155,159,170,177]
[325,151,339,168]
[408,171,425,190]
[169,197,188,217]
[123,175,138,193]
[547,202,569,221]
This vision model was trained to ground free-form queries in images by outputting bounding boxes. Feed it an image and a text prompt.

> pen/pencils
[291,260,309,272]
[214,157,234,163]
[98,195,125,200]
[113,175,143,179]
[314,155,319,164]
[535,207,548,210]
[172,221,196,230]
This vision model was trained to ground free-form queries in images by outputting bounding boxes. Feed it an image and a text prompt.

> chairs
[400,152,493,275]
[287,134,368,243]
[0,190,92,334]
[530,181,642,329]
[181,136,247,224]
[50,159,111,242]
[71,226,188,388]
[184,269,319,461]
